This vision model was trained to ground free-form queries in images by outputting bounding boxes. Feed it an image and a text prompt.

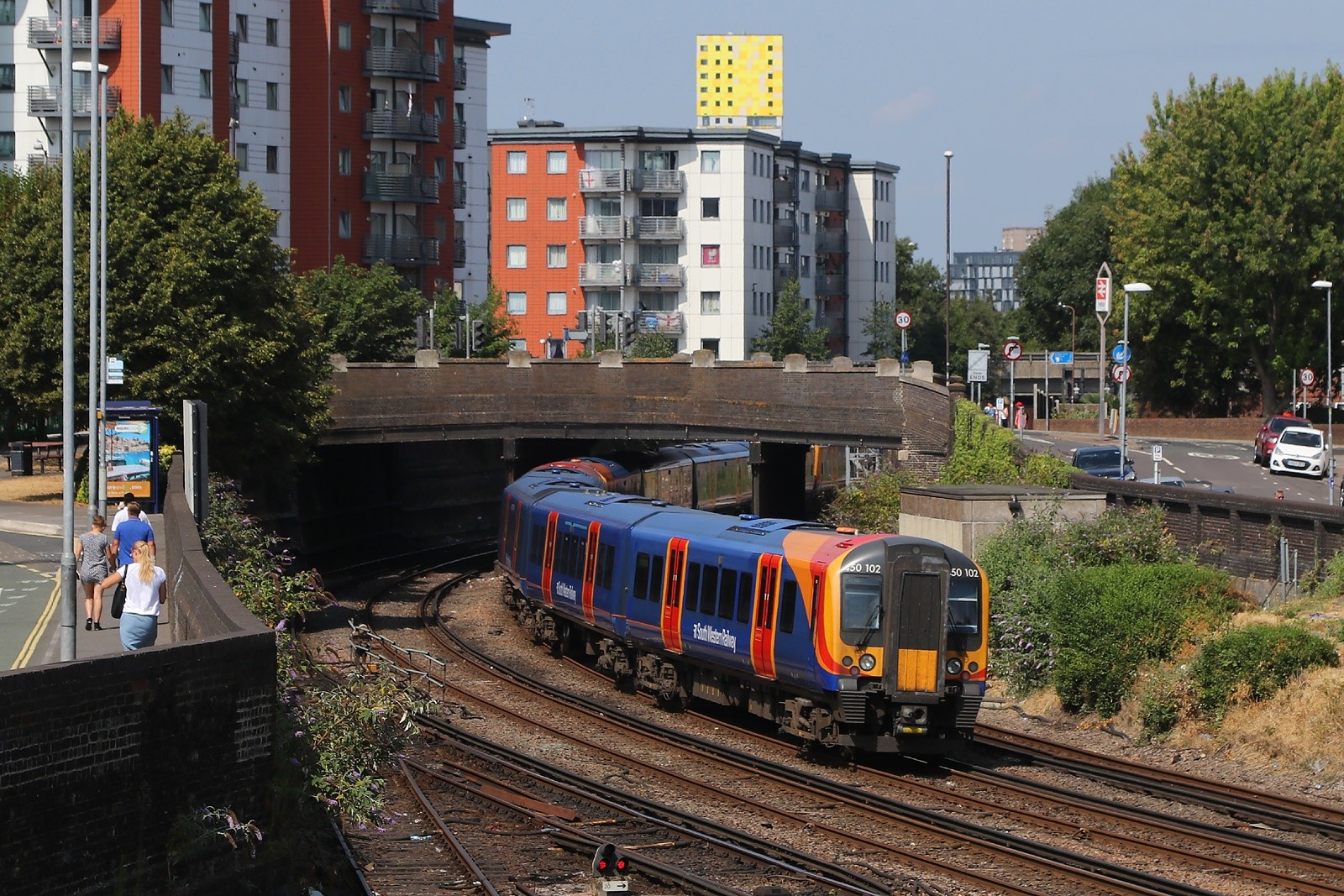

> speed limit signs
[1301,368,1315,386]
[894,311,911,329]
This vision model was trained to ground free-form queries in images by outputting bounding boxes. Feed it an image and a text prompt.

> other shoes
[93,622,102,630]
[86,618,92,630]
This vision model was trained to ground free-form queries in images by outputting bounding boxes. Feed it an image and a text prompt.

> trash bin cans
[11,441,33,476]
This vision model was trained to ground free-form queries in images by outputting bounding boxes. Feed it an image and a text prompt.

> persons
[76,515,116,630]
[984,402,996,419]
[1003,404,1008,428]
[111,493,154,532]
[110,501,156,573]
[101,540,167,653]
[1015,402,1027,430]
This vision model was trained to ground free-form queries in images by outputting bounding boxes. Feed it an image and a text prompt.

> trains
[497,440,990,764]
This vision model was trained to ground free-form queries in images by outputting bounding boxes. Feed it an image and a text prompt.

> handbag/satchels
[111,564,128,619]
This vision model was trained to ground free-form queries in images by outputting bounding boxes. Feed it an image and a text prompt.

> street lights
[1058,302,1075,403]
[943,149,955,386]
[1119,283,1152,481]
[1312,280,1335,506]
[977,343,991,406]
[1007,335,1020,432]
[68,62,112,526]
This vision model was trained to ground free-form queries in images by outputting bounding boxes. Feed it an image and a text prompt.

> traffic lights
[599,312,613,344]
[473,320,484,351]
[623,318,635,347]
[575,313,586,343]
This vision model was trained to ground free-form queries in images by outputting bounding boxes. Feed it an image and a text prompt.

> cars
[1269,426,1329,479]
[1140,476,1188,488]
[1071,444,1136,481]
[1253,416,1315,467]
